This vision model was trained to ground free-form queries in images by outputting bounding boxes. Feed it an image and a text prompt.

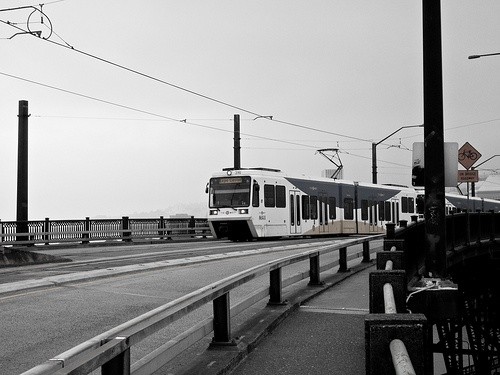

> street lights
[371,124,424,184]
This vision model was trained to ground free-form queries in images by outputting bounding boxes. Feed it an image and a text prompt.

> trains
[207,166,500,242]
